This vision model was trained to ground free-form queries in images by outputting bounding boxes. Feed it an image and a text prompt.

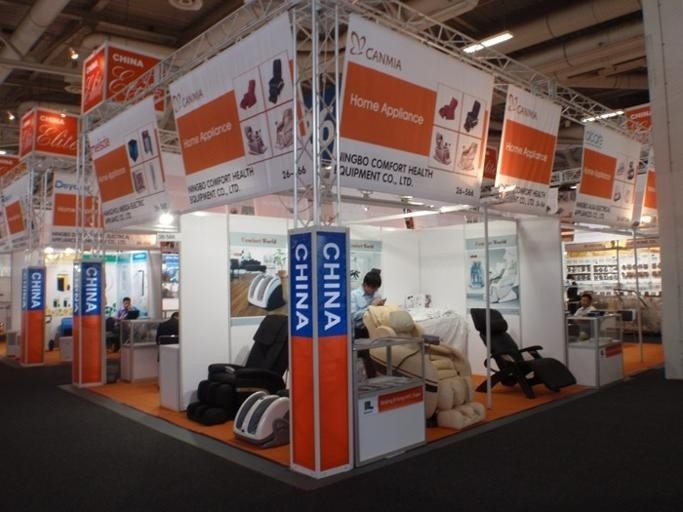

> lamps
[581,109,624,124]
[461,30,516,55]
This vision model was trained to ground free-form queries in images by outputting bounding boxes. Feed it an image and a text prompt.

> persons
[573,293,599,337]
[111,296,133,352]
[351,270,387,379]
[155,312,179,362]
[566,281,581,315]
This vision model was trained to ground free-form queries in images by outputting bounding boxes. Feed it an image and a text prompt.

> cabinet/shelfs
[48,312,466,479]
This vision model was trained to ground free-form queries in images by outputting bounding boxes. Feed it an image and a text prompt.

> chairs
[469,306,578,399]
[361,306,486,429]
